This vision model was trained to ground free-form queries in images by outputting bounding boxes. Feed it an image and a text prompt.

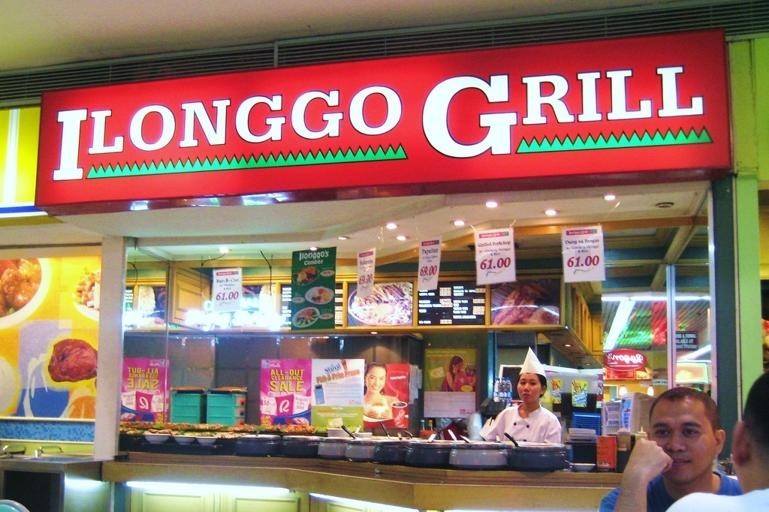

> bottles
[419,419,432,431]
[617,427,648,451]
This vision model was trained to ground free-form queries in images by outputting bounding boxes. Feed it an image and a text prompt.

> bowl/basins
[143,433,217,447]
[568,463,596,472]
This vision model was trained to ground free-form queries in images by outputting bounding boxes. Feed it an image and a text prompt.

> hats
[518,347,547,378]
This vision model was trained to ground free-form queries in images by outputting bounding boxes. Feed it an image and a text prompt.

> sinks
[0,455,29,459]
[22,455,95,463]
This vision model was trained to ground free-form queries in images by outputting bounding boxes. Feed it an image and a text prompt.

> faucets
[2,444,27,455]
[33,445,63,458]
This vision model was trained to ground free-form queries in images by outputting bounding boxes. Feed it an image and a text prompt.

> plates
[73,287,99,321]
[346,287,412,325]
[0,257,49,327]
[291,269,334,328]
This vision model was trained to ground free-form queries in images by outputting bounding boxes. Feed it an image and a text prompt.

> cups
[391,401,408,428]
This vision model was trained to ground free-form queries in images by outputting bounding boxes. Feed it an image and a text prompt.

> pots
[316,436,567,472]
[235,429,320,457]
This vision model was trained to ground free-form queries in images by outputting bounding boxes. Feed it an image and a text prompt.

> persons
[360,361,403,422]
[482,373,562,445]
[663,369,768,512]
[439,355,471,392]
[597,386,742,512]
